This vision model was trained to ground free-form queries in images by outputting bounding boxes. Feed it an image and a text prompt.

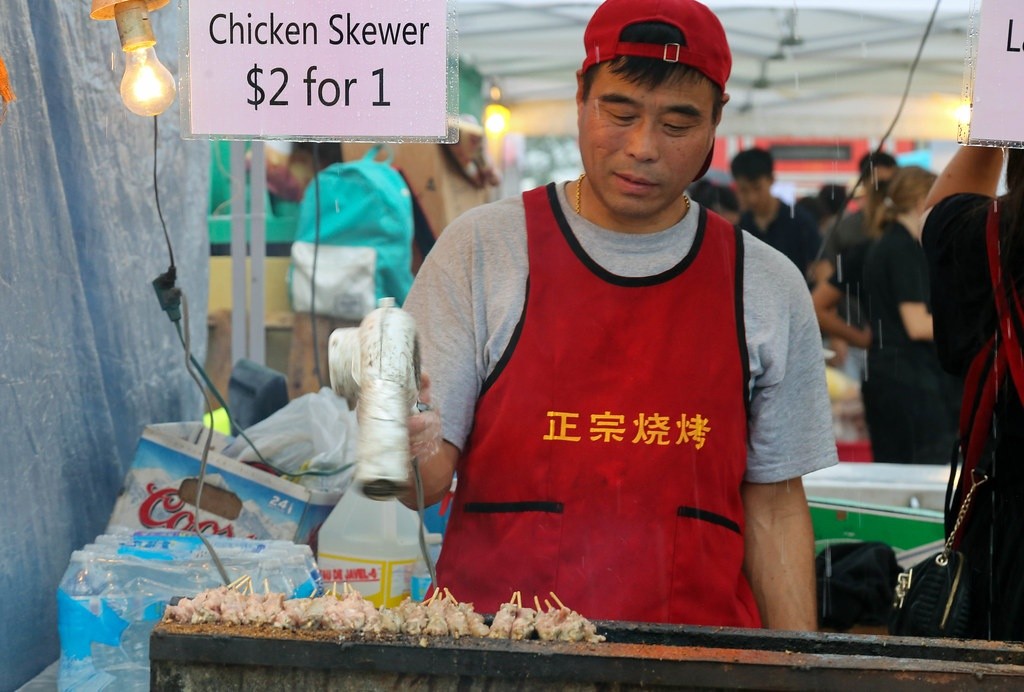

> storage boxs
[102,422,346,562]
[800,462,957,552]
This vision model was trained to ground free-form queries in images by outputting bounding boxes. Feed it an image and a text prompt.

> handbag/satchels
[225,386,361,492]
[812,541,906,632]
[888,548,993,639]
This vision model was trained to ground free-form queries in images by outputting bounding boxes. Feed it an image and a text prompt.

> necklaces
[576,174,690,215]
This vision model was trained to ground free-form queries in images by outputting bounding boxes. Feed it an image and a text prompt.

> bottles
[317,481,428,611]
[58,535,324,692]
[410,534,444,604]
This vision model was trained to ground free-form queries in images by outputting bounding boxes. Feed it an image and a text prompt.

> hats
[583,0,732,182]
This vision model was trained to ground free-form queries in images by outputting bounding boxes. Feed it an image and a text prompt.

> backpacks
[288,143,415,320]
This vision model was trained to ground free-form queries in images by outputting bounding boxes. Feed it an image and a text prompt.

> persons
[690,144,1024,641]
[400,0,841,631]
